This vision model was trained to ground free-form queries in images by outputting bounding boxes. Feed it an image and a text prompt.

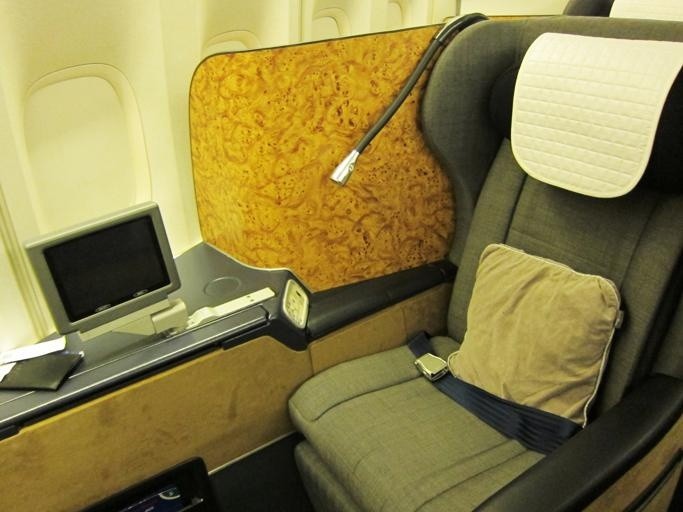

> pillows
[446,243,621,431]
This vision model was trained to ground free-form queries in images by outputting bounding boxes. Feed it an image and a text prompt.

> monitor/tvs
[23,200,181,335]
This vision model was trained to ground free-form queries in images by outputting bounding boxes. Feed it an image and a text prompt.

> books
[0,350,83,390]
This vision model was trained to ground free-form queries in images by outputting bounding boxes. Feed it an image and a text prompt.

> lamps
[328,13,486,186]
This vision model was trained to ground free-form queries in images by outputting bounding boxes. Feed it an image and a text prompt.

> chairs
[286,15,682,511]
